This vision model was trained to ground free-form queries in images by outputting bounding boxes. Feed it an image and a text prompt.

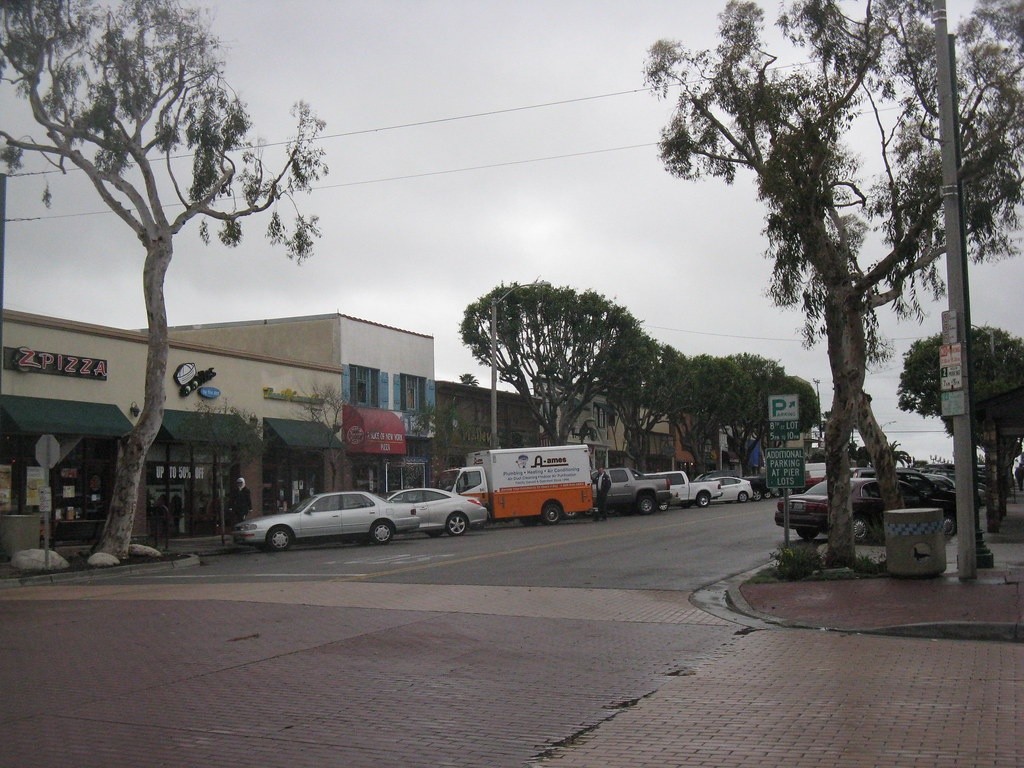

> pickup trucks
[592,467,671,515]
[634,471,724,509]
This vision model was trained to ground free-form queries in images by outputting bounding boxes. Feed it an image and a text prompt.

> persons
[591,468,611,521]
[228,478,253,524]
[1015,463,1024,491]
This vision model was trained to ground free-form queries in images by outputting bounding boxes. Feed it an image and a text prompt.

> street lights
[489,280,553,450]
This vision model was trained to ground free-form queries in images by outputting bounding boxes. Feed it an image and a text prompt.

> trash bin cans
[884,508,947,577]
[1,514,40,556]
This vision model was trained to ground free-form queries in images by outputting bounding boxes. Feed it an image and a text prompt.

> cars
[381,488,487,539]
[775,478,940,543]
[704,476,775,502]
[233,489,419,551]
[806,461,985,513]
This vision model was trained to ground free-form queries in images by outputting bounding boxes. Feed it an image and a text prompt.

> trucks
[436,444,594,525]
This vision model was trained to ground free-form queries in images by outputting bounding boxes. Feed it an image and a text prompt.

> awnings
[0,394,407,455]
